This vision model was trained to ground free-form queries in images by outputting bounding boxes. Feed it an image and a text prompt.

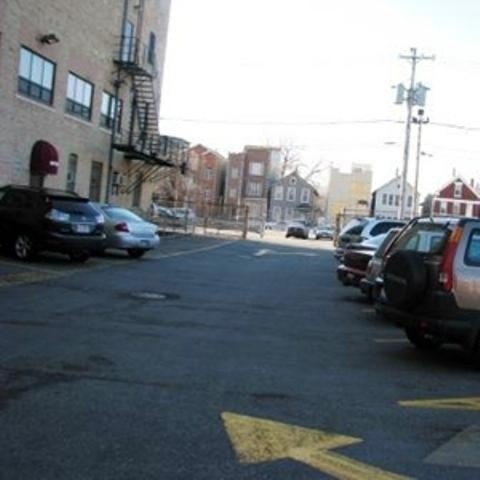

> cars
[285,222,334,239]
[90,200,161,258]
[157,203,196,220]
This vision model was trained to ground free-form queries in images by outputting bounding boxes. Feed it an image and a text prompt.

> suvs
[333,213,480,349]
[0,182,108,263]
